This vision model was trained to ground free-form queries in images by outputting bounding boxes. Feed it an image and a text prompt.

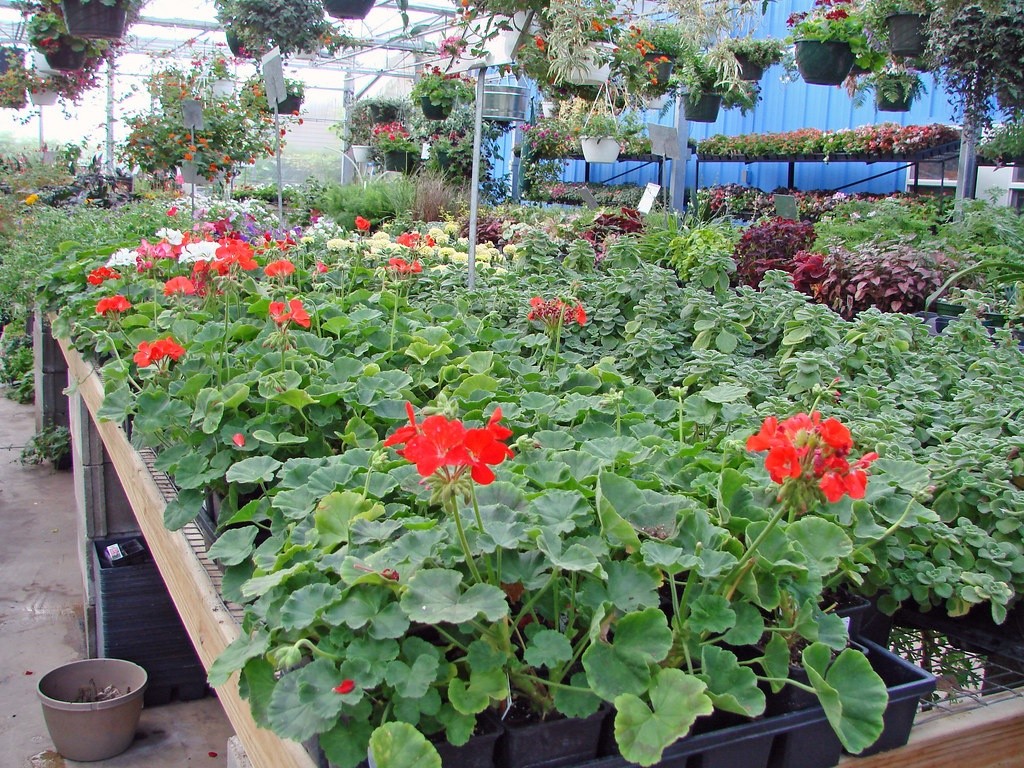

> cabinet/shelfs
[513,146,690,218]
[690,139,975,234]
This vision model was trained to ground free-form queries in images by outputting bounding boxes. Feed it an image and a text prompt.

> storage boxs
[91,538,217,710]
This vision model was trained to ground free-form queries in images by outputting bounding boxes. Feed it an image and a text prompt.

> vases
[442,623,934,768]
[877,75,913,113]
[268,91,302,116]
[884,14,929,56]
[565,42,619,85]
[641,51,674,83]
[421,95,452,121]
[793,40,854,87]
[60,2,129,40]
[680,93,721,122]
[369,103,398,124]
[37,659,146,760]
[293,42,319,60]
[384,150,416,171]
[225,25,269,58]
[28,79,60,106]
[579,135,621,164]
[734,52,763,80]
[322,0,375,20]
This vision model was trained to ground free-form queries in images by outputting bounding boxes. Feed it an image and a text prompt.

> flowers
[351,146,374,162]
[0,0,1024,768]
